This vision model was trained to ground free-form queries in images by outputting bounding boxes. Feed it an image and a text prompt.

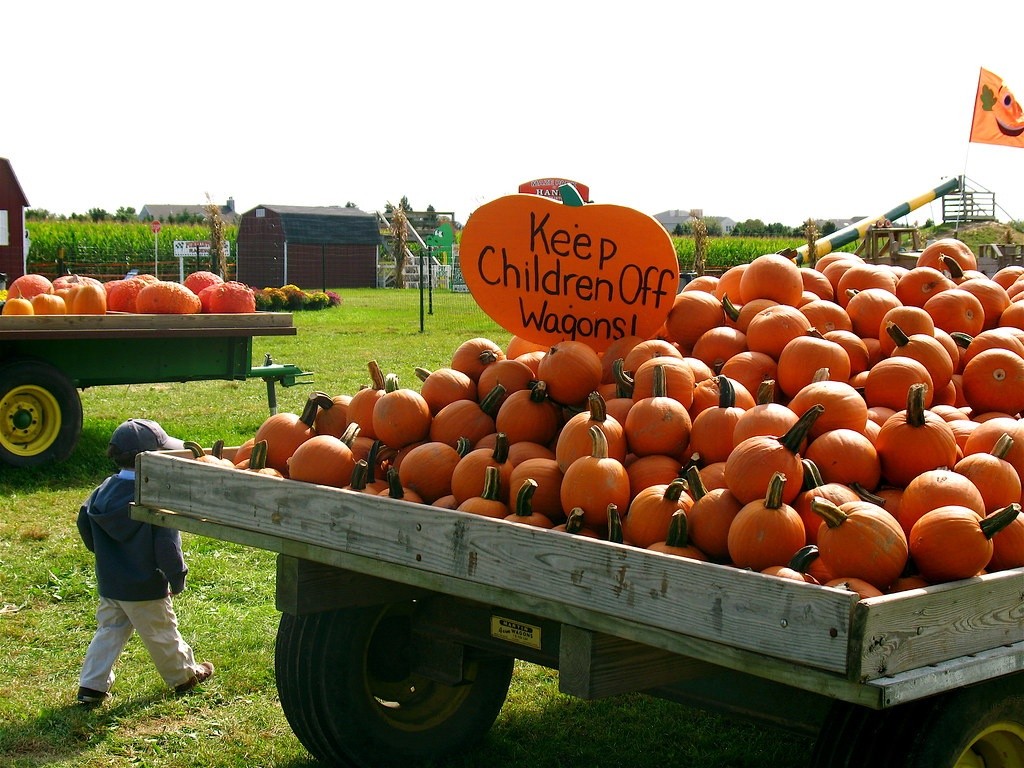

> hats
[108,418,185,460]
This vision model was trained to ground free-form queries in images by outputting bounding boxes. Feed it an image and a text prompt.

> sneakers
[77,687,113,702]
[175,662,214,693]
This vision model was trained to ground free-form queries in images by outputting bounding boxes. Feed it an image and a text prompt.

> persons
[76,418,214,707]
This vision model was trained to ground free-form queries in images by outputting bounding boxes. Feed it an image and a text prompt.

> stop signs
[151,220,162,233]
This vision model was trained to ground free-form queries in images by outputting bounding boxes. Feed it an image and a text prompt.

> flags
[966,66,1024,148]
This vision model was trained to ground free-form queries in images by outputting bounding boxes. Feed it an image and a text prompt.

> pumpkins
[2,269,256,314]
[185,239,1024,598]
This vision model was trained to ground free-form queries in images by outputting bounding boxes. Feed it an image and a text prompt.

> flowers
[254,289,273,311]
[304,292,320,311]
[287,290,306,313]
[313,292,329,310]
[270,288,288,312]
[325,291,343,308]
[281,284,301,296]
